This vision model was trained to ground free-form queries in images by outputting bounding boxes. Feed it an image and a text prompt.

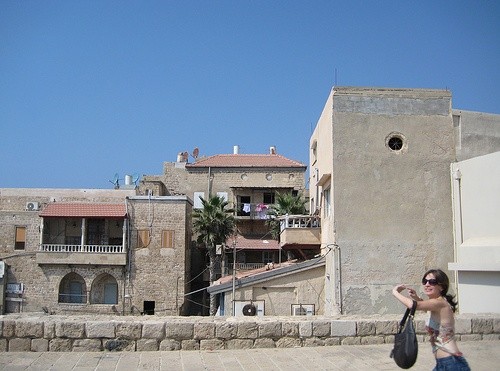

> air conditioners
[294,305,314,316]
[233,301,264,317]
[25,202,38,210]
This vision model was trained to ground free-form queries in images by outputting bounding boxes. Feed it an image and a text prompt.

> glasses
[422,278,439,286]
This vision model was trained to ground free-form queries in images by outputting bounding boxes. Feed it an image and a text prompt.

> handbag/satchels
[393,318,419,369]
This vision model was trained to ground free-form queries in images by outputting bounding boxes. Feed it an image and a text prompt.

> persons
[393,269,470,371]
